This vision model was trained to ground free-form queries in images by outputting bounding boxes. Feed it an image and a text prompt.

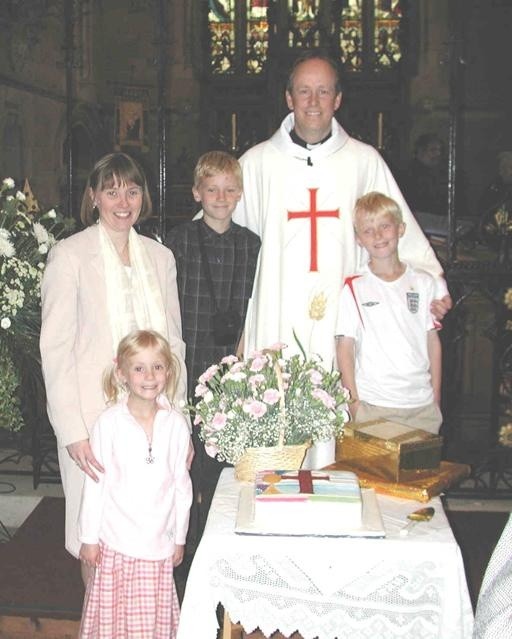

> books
[320,456,474,504]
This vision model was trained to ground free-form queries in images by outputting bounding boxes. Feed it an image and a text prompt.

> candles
[378,113,382,148]
[232,114,236,149]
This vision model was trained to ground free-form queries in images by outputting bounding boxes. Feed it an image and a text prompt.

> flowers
[179,343,352,465]
[0,175,77,432]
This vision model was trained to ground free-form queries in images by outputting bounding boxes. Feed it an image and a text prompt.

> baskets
[233,439,310,481]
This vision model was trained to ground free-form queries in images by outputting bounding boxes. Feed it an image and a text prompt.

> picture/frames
[114,96,150,153]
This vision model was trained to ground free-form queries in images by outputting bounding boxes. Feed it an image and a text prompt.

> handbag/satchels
[212,311,241,345]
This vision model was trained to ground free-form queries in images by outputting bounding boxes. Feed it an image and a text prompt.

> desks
[175,468,474,639]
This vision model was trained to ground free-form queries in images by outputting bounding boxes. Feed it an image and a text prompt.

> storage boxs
[335,418,444,483]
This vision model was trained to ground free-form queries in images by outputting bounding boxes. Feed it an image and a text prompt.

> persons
[475,151,511,253]
[396,133,465,215]
[161,150,264,559]
[37,150,197,590]
[189,48,456,374]
[329,194,447,441]
[76,325,197,639]
